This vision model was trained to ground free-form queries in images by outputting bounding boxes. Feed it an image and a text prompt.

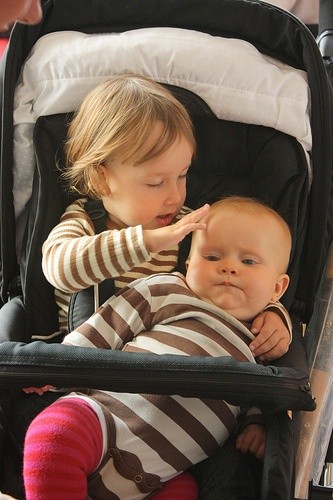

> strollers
[0,1,333,500]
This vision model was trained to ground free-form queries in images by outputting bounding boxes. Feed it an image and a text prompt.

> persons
[40,73,292,500]
[0,0,42,33]
[21,195,292,500]
